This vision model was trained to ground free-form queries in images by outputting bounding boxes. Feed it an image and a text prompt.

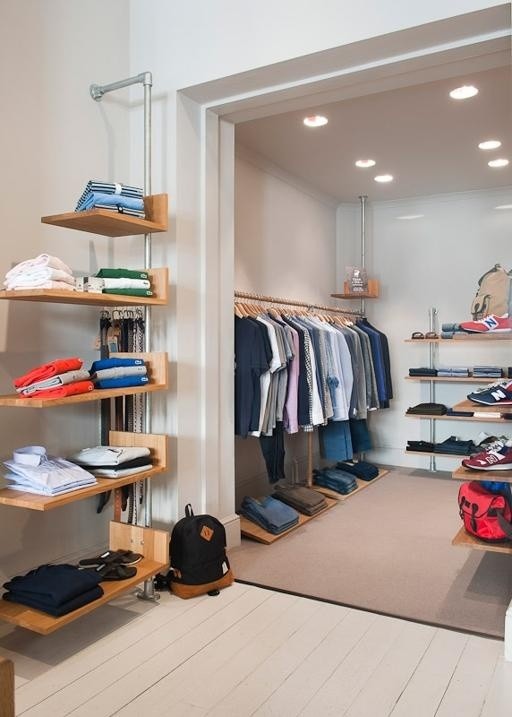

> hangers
[235,289,388,339]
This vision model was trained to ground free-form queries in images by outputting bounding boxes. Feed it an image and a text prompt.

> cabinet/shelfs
[0,194,169,635]
[404,333,512,555]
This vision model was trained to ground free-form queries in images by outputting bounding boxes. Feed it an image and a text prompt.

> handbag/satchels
[458,480,512,543]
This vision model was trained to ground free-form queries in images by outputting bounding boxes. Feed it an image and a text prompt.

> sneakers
[459,313,512,333]
[462,431,512,471]
[467,379,512,405]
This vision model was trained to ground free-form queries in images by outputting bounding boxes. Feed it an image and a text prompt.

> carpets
[226,461,512,639]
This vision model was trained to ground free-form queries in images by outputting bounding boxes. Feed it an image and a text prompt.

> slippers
[78,549,143,581]
[412,332,439,339]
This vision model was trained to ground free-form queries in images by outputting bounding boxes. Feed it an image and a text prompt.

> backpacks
[169,503,233,599]
[471,263,512,321]
